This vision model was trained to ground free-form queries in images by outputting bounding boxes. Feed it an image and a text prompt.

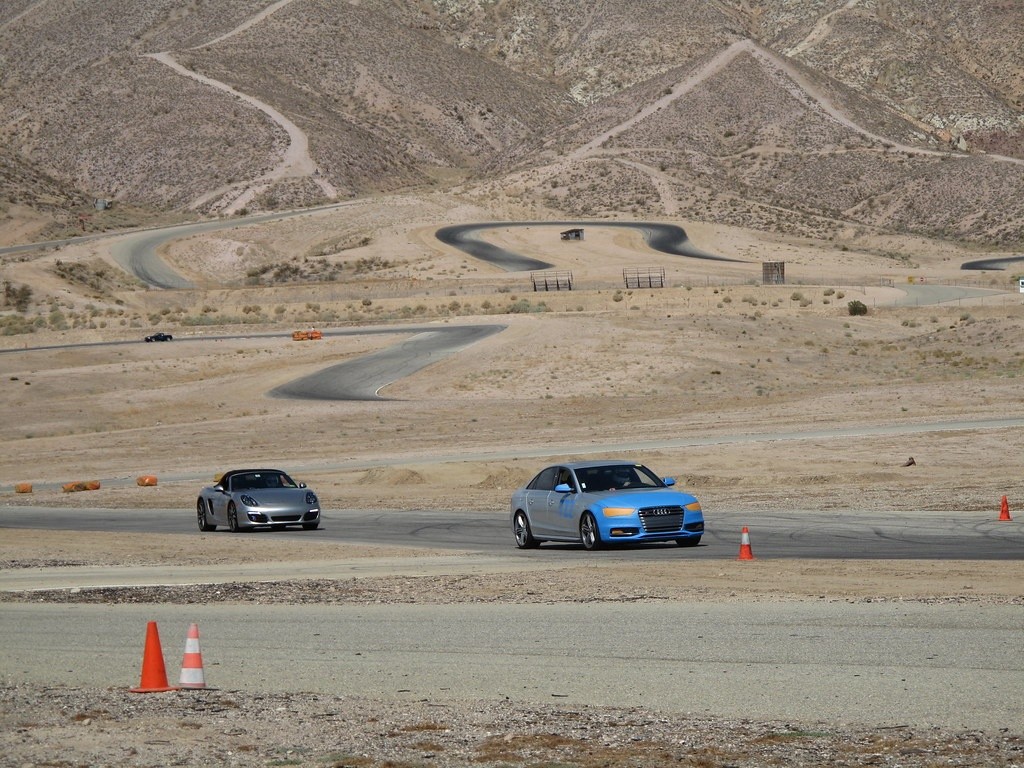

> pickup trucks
[145,332,173,343]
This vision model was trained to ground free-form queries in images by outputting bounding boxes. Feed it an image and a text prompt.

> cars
[510,459,705,552]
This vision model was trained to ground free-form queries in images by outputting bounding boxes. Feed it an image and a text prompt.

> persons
[607,468,633,491]
[262,474,278,488]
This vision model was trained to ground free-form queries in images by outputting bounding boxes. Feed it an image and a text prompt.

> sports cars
[197,468,321,533]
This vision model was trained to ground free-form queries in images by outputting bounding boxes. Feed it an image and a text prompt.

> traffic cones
[735,525,758,562]
[175,624,219,692]
[127,620,179,693]
[996,494,1013,521]
